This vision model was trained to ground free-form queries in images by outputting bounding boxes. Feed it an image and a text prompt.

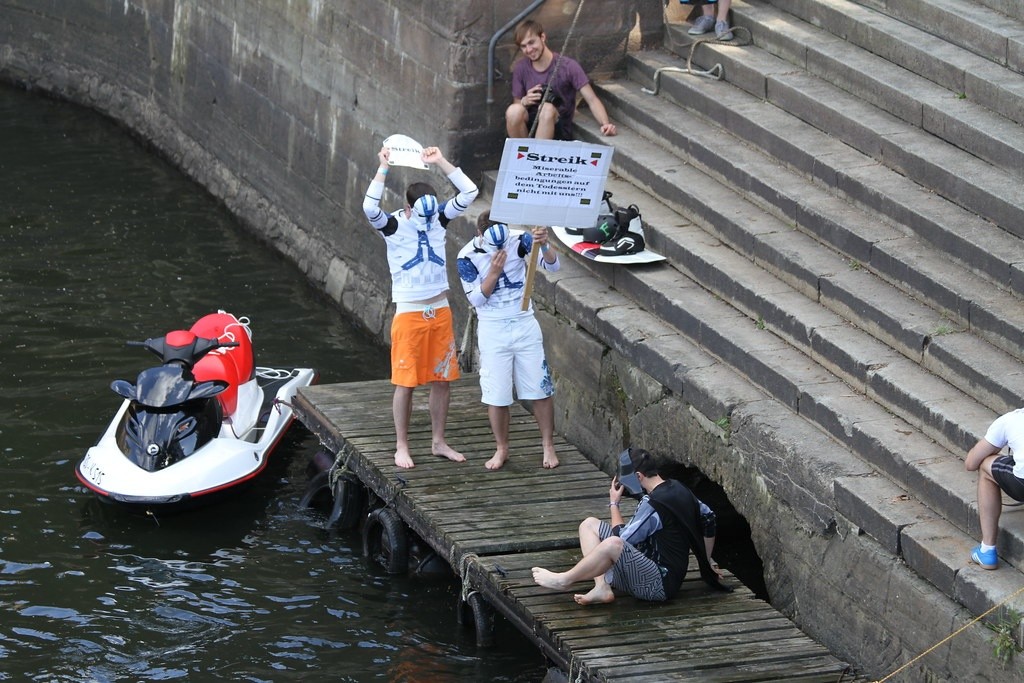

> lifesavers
[361,507,411,577]
[455,585,493,648]
[298,469,368,536]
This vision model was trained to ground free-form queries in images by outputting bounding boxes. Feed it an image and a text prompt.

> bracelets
[608,502,619,508]
[541,245,550,252]
[377,168,387,175]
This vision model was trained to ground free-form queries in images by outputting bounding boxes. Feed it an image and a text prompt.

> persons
[531,449,725,604]
[505,21,617,140]
[457,210,561,469]
[363,146,478,469]
[965,408,1024,570]
[680,0,733,41]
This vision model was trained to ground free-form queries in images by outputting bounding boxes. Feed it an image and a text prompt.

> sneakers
[566,189,613,235]
[599,205,646,255]
[971,544,998,570]
[715,20,733,40]
[687,15,716,35]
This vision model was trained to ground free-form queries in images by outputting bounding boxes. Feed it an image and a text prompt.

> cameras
[534,84,562,107]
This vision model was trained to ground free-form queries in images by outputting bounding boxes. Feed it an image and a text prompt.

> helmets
[583,213,616,243]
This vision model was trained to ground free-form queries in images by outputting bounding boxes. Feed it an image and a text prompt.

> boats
[75,312,321,520]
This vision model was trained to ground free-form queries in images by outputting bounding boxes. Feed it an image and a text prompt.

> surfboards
[550,222,668,267]
